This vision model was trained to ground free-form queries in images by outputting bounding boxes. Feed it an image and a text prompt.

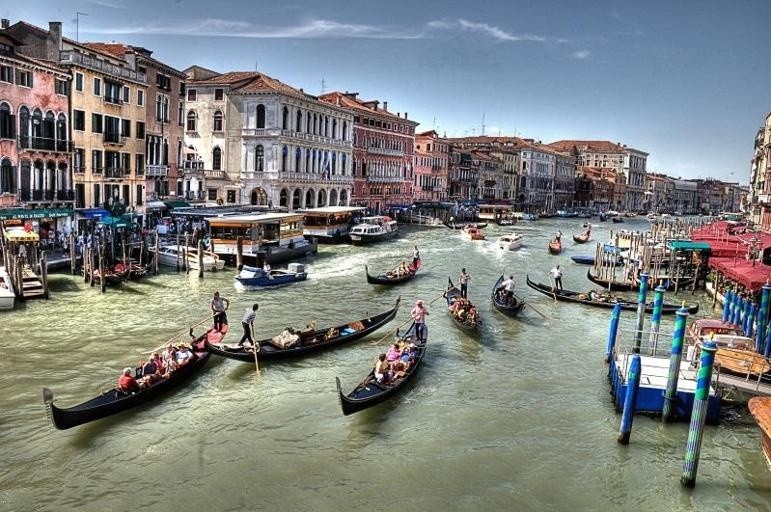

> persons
[411,245,420,269]
[263,260,271,276]
[18,228,111,266]
[210,292,230,332]
[554,230,563,243]
[550,265,564,291]
[239,304,259,346]
[451,301,476,321]
[410,300,429,344]
[459,268,471,298]
[374,344,416,383]
[117,341,194,394]
[387,261,408,279]
[502,275,515,307]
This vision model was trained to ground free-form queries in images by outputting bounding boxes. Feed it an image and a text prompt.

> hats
[416,300,423,305]
[177,341,190,348]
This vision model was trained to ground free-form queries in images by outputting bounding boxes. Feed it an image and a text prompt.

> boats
[77,254,131,286]
[364,248,422,288]
[446,275,481,340]
[206,297,403,363]
[440,209,683,266]
[586,269,703,292]
[748,394,771,461]
[688,318,771,380]
[349,213,400,243]
[43,312,229,431]
[148,246,227,273]
[490,273,526,320]
[526,271,699,316]
[238,262,309,288]
[0,268,17,310]
[337,315,429,419]
